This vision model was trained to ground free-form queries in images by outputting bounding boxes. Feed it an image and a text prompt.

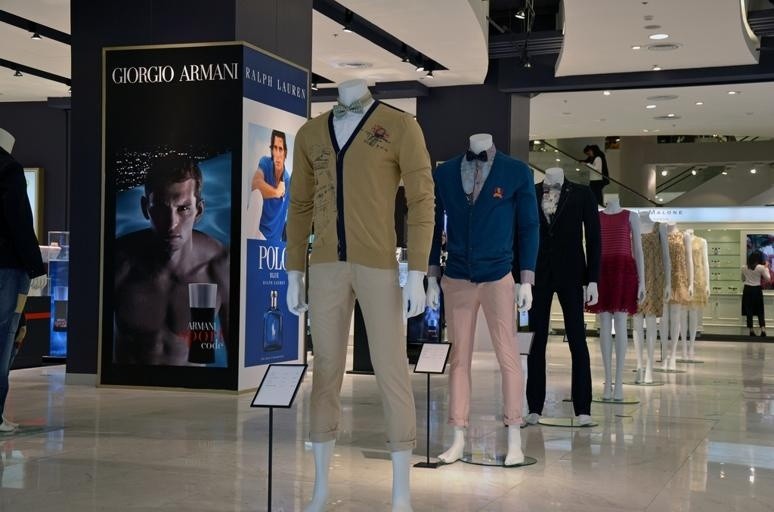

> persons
[244,129,289,242]
[740,251,771,336]
[630,211,671,382]
[284,78,435,511]
[678,228,710,360]
[658,222,693,371]
[578,145,609,190]
[115,154,230,368]
[430,134,543,467]
[580,144,604,206]
[512,166,600,425]
[0,127,48,433]
[582,193,646,400]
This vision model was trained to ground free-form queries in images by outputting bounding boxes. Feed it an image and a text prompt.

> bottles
[428,318,437,339]
[261,291,283,354]
[53,285,67,332]
[187,282,218,363]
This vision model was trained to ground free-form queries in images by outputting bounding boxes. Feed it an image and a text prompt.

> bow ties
[333,99,364,120]
[466,151,488,162]
[542,183,561,191]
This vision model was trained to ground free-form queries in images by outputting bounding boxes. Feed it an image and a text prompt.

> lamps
[342,13,434,78]
[13,70,23,76]
[515,0,535,68]
[311,83,319,91]
[31,32,42,40]
